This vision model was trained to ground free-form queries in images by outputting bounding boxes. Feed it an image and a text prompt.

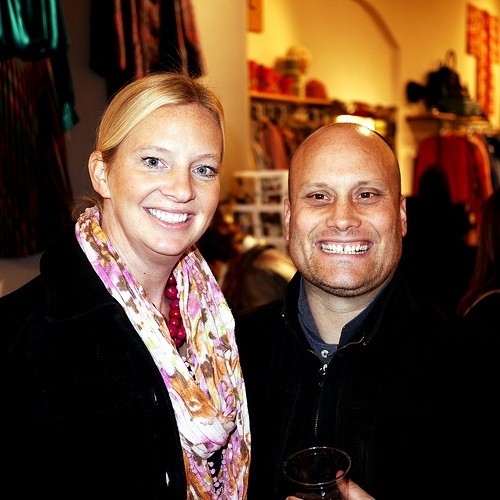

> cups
[282,447,351,499]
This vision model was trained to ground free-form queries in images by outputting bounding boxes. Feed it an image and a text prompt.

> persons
[0,75,252,500]
[197,227,297,313]
[236,122,500,500]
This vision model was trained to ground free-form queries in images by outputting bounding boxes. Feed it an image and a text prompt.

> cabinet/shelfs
[250,91,397,212]
[231,169,288,246]
[406,108,500,291]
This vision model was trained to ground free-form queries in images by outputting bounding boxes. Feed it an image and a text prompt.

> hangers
[252,103,391,129]
[433,120,495,137]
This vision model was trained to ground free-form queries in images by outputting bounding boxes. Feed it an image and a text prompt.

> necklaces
[161,276,186,348]
